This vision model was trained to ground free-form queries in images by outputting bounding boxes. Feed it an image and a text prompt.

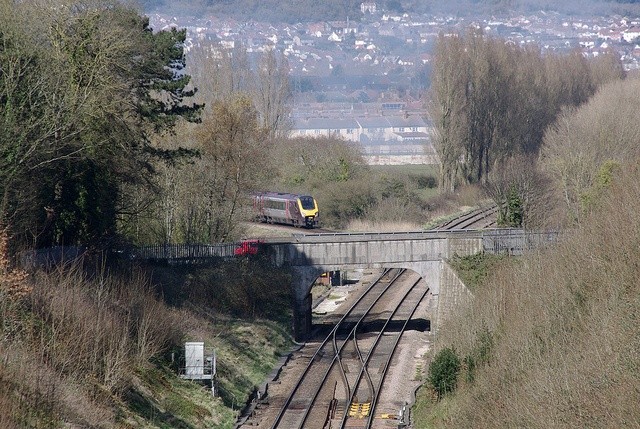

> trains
[249,191,320,230]
